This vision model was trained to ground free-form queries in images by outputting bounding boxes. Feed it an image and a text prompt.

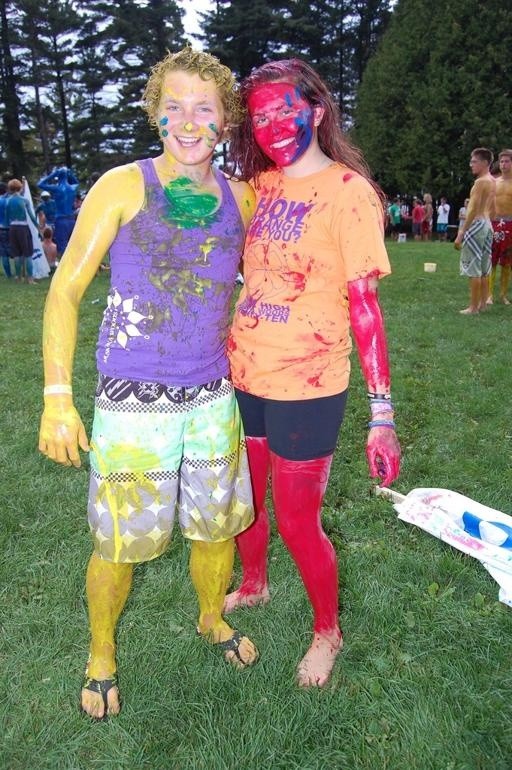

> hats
[40,191,50,196]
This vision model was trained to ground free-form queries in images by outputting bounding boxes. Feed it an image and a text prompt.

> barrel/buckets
[423,262,437,273]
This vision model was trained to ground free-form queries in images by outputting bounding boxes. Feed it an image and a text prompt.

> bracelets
[364,391,397,431]
[41,383,74,399]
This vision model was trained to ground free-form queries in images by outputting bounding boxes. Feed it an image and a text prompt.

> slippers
[81,652,121,722]
[195,625,259,669]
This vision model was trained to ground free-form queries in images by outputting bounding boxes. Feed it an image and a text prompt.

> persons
[484,149,511,309]
[382,191,470,244]
[489,160,502,179]
[35,48,353,722]
[219,55,405,693]
[0,165,111,286]
[452,146,499,317]
